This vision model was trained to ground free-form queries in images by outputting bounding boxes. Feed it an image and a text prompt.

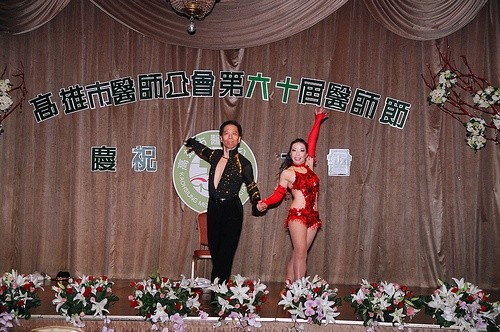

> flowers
[429,69,458,107]
[127,271,210,332]
[52,274,121,332]
[420,277,500,332]
[0,79,14,111]
[493,111,500,131]
[277,276,342,332]
[205,275,270,331]
[466,116,487,153]
[0,269,45,331]
[469,85,500,109]
[344,280,422,331]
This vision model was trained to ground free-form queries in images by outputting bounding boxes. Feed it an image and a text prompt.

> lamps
[170,0,220,34]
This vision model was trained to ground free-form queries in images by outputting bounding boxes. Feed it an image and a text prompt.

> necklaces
[222,155,228,160]
[292,161,305,167]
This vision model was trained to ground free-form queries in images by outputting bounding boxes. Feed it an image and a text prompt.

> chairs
[191,212,211,282]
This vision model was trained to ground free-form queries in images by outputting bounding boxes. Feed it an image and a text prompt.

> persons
[258,105,328,295]
[184,120,266,296]
